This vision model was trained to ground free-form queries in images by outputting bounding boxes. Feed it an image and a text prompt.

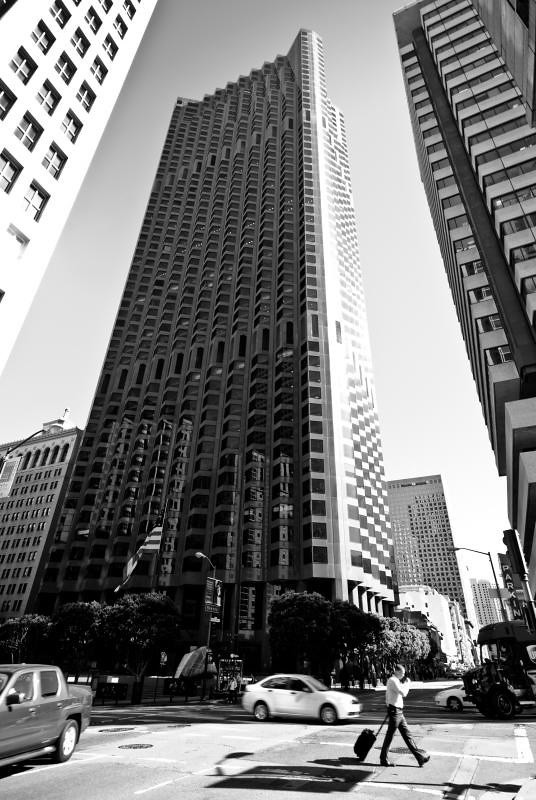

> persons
[379,664,431,767]
[225,672,241,704]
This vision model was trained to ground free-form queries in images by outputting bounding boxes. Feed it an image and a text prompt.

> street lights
[447,547,508,622]
[194,552,216,702]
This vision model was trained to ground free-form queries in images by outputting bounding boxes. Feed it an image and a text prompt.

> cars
[0,663,93,767]
[242,673,364,724]
[434,685,476,713]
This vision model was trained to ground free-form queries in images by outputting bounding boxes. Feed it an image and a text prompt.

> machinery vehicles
[461,620,536,719]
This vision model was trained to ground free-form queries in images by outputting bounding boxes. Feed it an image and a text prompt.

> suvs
[446,670,463,679]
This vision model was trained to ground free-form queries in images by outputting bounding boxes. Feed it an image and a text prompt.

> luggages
[354,711,389,761]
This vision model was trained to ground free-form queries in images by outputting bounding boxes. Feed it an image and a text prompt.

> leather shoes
[418,755,430,767]
[381,759,396,767]
